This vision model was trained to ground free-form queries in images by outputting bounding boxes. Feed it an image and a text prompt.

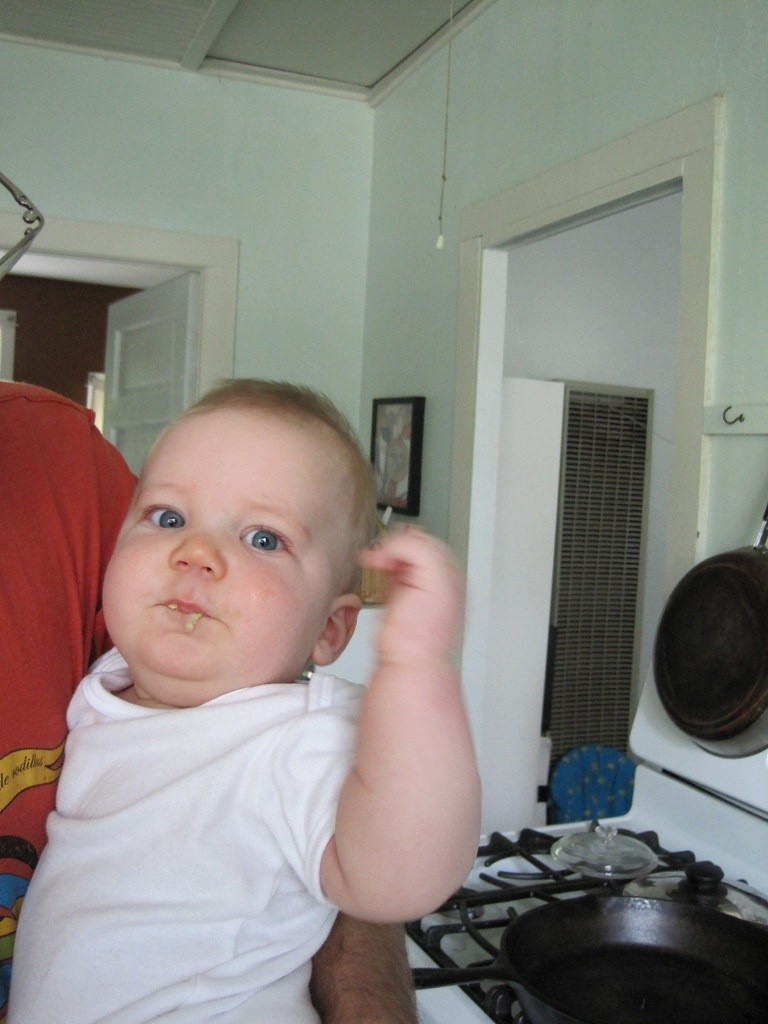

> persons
[380,413,411,499]
[0,379,482,1024]
[0,382,416,1024]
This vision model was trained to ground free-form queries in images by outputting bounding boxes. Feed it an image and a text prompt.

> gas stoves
[388,763,768,1024]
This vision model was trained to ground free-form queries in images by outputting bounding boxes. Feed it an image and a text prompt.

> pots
[410,891,768,1024]
[650,494,768,761]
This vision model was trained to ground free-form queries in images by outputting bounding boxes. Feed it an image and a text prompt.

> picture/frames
[370,396,426,518]
